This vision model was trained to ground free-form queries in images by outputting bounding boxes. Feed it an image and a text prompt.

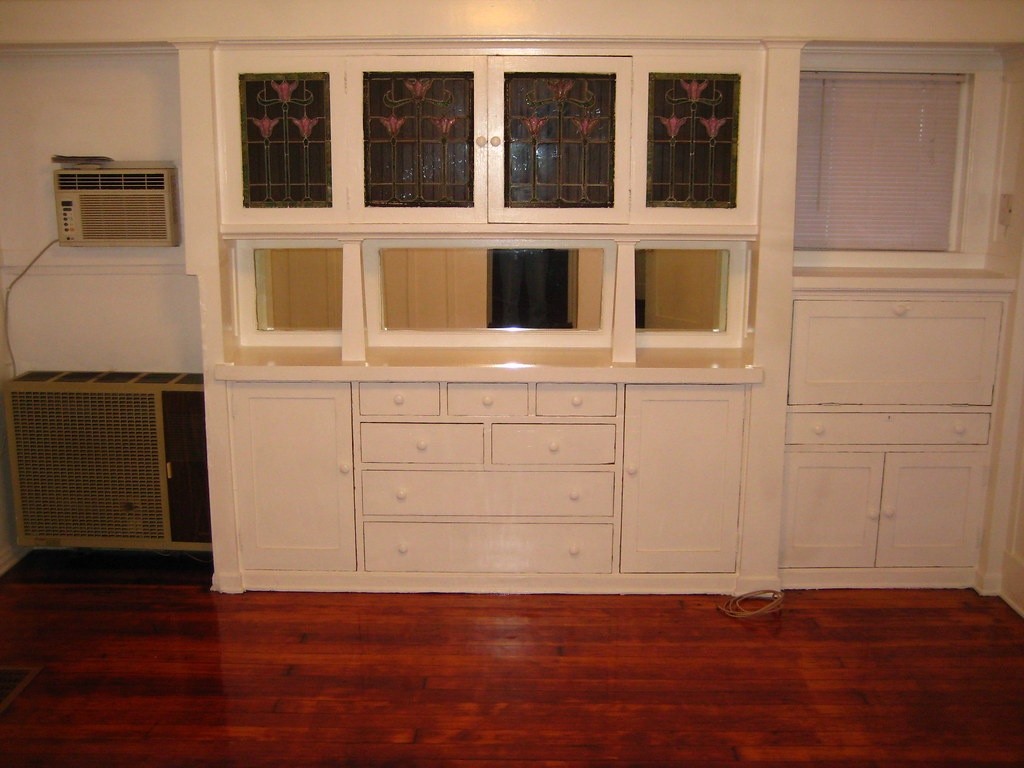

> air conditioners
[52,169,177,248]
[0,370,212,553]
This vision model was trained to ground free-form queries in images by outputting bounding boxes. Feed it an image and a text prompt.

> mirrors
[253,247,342,332]
[379,247,604,332]
[633,248,730,333]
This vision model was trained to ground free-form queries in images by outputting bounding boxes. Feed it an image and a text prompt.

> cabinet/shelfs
[358,382,617,576]
[785,292,1005,408]
[620,382,752,575]
[630,50,766,242]
[784,410,990,446]
[225,383,358,573]
[213,48,348,240]
[347,49,634,240]
[778,451,991,567]
